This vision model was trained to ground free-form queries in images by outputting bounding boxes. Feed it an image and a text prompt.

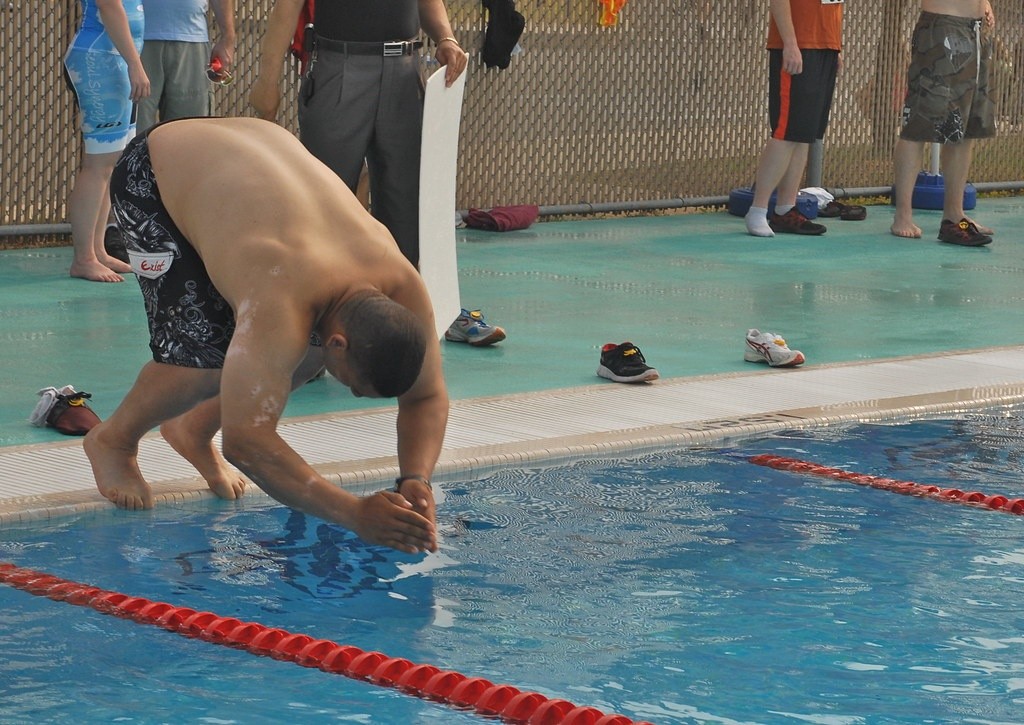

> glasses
[205,62,235,86]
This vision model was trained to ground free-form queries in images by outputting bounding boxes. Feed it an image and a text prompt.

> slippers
[818,200,866,220]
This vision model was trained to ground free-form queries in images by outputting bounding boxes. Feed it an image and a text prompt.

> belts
[316,35,424,57]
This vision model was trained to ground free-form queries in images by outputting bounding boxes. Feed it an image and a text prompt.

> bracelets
[437,37,459,45]
[393,474,433,493]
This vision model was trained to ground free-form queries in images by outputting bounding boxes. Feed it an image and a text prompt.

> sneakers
[104,226,130,264]
[769,206,826,235]
[937,218,992,245]
[744,329,805,367]
[445,309,506,345]
[596,342,659,383]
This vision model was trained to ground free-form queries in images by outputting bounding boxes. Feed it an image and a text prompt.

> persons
[59,1,236,283]
[743,0,844,237]
[83,116,450,555]
[890,1,995,239]
[248,0,467,274]
